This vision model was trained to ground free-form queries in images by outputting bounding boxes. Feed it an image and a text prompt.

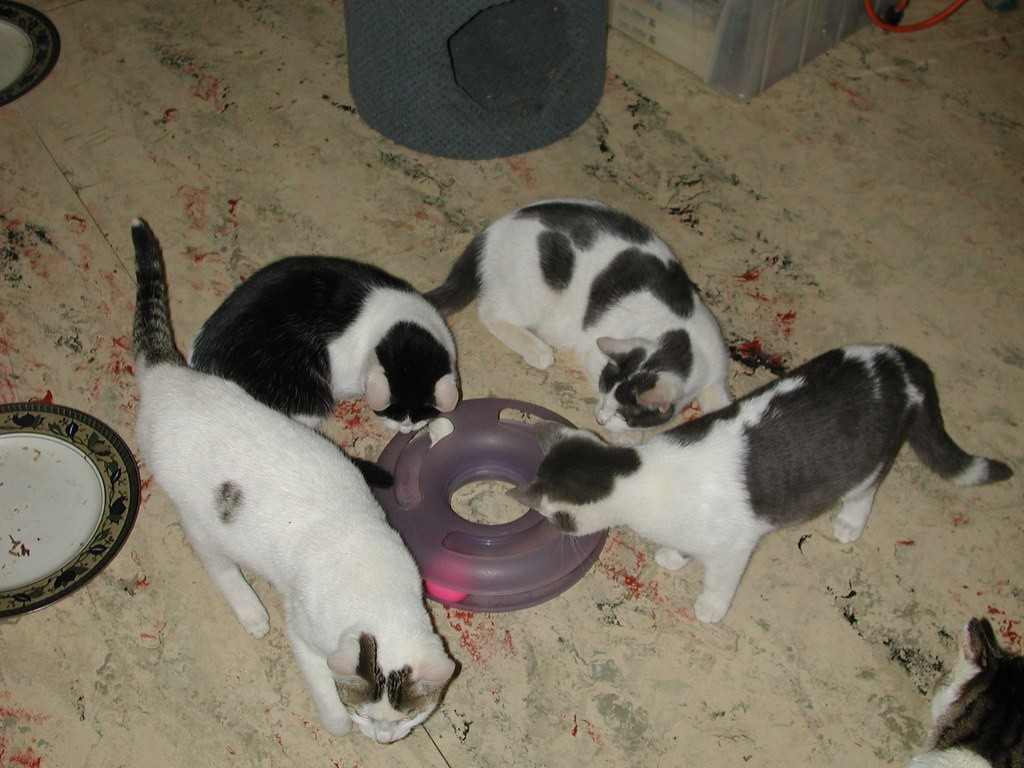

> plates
[2,401,140,619]
[0,0,60,105]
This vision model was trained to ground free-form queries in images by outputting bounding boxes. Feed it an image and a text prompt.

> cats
[129,199,1023,768]
